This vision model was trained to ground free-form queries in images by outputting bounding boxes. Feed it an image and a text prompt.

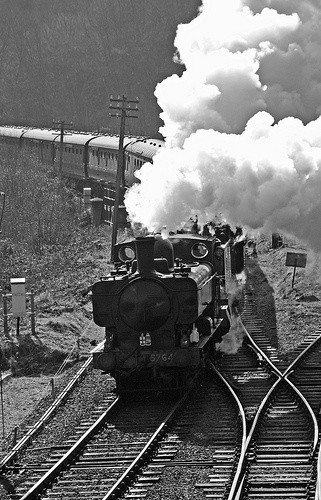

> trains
[1,123,249,396]
